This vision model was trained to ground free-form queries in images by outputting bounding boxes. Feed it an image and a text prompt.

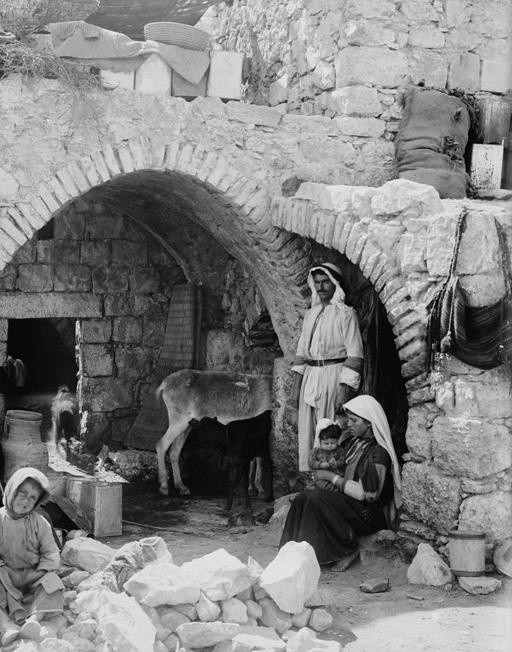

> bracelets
[331,473,340,484]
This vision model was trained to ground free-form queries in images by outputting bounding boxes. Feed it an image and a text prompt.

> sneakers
[1,628,19,648]
[21,618,41,639]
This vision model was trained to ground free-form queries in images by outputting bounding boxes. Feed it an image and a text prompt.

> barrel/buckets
[448,520,487,577]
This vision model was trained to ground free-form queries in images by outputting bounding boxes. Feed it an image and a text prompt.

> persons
[0,468,65,645]
[278,393,404,572]
[308,416,349,494]
[293,263,365,474]
